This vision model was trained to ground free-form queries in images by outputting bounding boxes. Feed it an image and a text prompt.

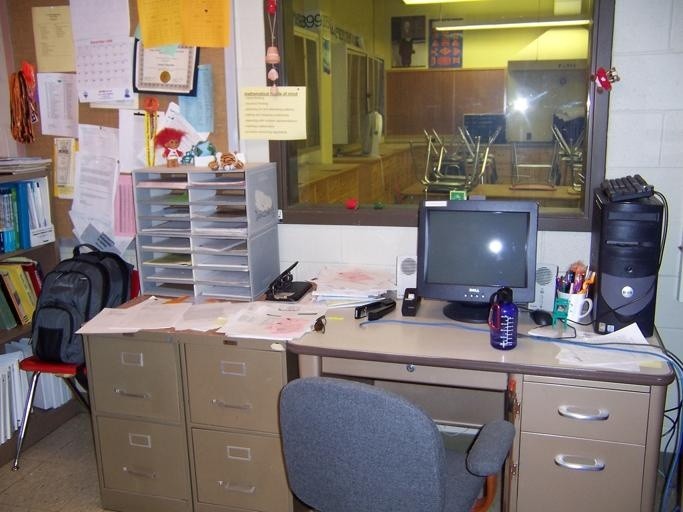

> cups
[314,315,327,333]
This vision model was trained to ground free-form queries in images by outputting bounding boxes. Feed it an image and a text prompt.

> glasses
[431,2,591,31]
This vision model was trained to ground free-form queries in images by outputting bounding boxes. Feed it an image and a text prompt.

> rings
[558,265,594,293]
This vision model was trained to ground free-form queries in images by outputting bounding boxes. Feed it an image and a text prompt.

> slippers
[31,244,130,364]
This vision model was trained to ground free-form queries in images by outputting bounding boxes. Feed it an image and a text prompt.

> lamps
[419,124,586,193]
[277,376,516,512]
[12,268,141,469]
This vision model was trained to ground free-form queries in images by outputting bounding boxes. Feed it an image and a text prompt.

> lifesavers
[588,187,663,337]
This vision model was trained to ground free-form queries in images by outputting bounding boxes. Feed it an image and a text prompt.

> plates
[0,168,80,468]
[132,162,281,302]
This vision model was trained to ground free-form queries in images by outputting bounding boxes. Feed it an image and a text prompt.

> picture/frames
[79,292,675,512]
[401,182,582,208]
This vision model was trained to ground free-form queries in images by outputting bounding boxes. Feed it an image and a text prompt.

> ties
[416,200,538,324]
[463,113,506,144]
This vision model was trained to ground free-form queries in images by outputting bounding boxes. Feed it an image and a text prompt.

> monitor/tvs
[0,158,75,445]
[312,263,385,310]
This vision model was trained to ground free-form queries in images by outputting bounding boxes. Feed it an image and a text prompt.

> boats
[396,255,416,300]
[528,262,559,313]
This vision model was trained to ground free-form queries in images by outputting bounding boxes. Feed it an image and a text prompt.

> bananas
[601,174,654,201]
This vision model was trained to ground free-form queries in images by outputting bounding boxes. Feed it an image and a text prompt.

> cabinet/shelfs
[488,288,519,350]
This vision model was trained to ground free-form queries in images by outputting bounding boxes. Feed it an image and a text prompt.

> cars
[554,289,593,326]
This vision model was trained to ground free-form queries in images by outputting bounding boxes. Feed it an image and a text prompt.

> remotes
[531,309,553,325]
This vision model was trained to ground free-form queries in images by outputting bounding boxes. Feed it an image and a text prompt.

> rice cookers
[355,298,395,323]
